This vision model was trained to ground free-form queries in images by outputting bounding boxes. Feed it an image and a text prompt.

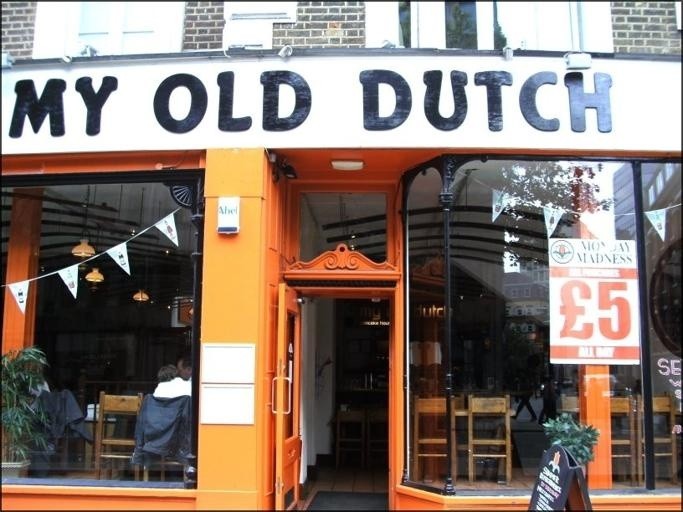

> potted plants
[2,345,53,480]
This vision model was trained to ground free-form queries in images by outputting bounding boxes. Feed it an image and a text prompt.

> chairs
[557,392,677,489]
[334,404,387,470]
[412,390,513,486]
[95,391,144,482]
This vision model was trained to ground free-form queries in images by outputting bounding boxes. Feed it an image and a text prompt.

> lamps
[69,185,106,283]
[329,160,366,172]
[133,288,151,301]
[283,164,296,180]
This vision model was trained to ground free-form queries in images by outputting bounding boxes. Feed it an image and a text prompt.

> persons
[134,364,195,466]
[513,392,537,422]
[538,376,557,424]
[176,356,193,381]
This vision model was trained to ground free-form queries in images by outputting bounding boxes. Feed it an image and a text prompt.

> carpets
[306,490,388,510]
